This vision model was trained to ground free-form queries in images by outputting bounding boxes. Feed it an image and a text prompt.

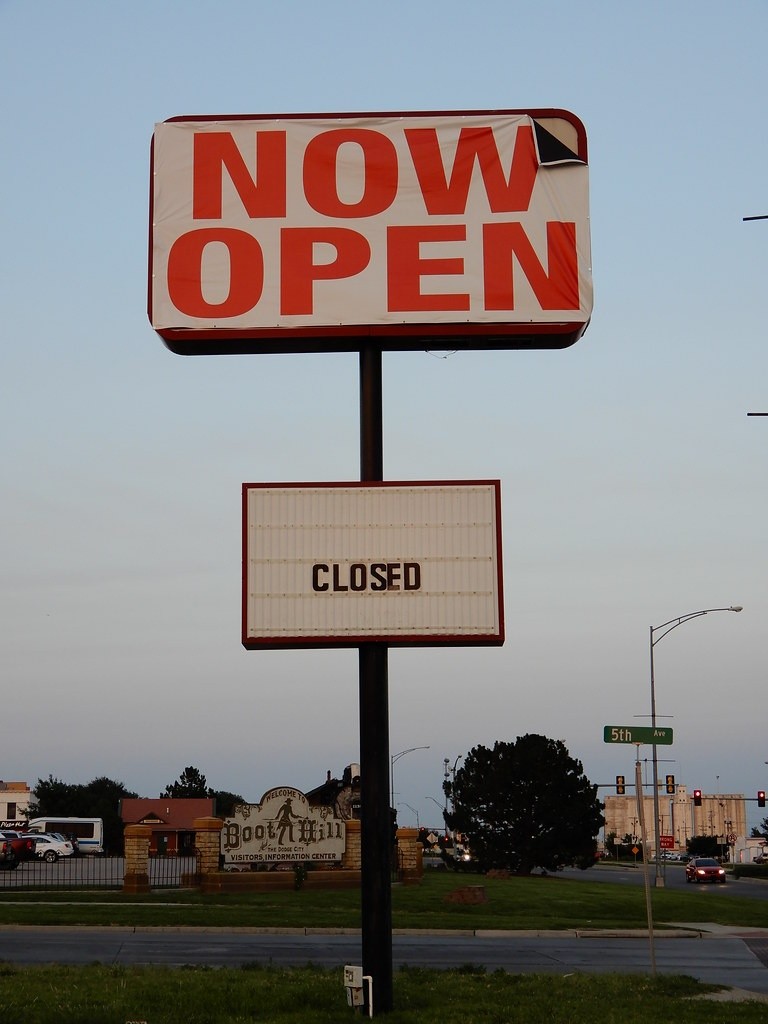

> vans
[27,817,104,858]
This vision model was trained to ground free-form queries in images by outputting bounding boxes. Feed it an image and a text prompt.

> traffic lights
[757,790,765,807]
[694,790,702,806]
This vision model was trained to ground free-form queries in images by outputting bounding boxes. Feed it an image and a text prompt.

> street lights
[452,753,463,779]
[649,605,744,889]
[397,802,419,827]
[424,796,448,828]
[391,745,431,809]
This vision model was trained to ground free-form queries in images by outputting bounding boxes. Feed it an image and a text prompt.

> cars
[660,852,722,864]
[0,830,80,871]
[686,858,726,884]
[754,852,768,864]
